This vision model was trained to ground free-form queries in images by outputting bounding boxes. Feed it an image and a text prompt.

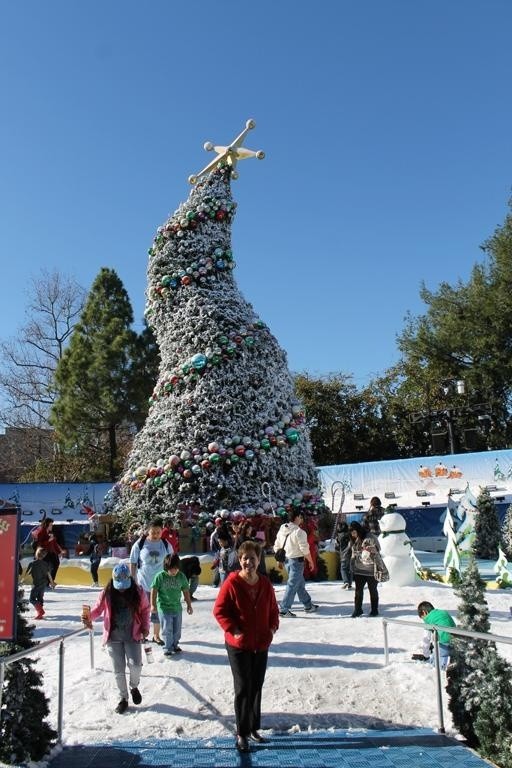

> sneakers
[304,603,319,613]
[130,687,142,704]
[115,698,128,714]
[164,647,182,655]
[279,611,296,618]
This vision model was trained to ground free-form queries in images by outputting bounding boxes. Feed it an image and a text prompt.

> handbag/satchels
[373,554,389,582]
[273,549,287,563]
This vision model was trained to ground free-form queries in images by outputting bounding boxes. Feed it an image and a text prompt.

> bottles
[144,638,154,664]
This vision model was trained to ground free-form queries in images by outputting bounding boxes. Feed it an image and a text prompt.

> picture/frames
[1,508,21,642]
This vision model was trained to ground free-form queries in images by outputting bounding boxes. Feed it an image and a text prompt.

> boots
[34,601,45,619]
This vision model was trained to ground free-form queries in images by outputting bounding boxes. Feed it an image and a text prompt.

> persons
[213,541,280,751]
[273,508,319,616]
[80,535,102,586]
[110,536,125,548]
[130,520,202,655]
[210,517,267,588]
[337,496,384,617]
[417,601,456,671]
[26,518,62,619]
[81,564,151,714]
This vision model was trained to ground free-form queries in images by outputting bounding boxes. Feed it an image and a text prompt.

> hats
[112,563,131,590]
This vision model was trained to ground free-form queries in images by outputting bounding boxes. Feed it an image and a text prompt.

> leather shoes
[237,735,249,751]
[249,730,266,744]
